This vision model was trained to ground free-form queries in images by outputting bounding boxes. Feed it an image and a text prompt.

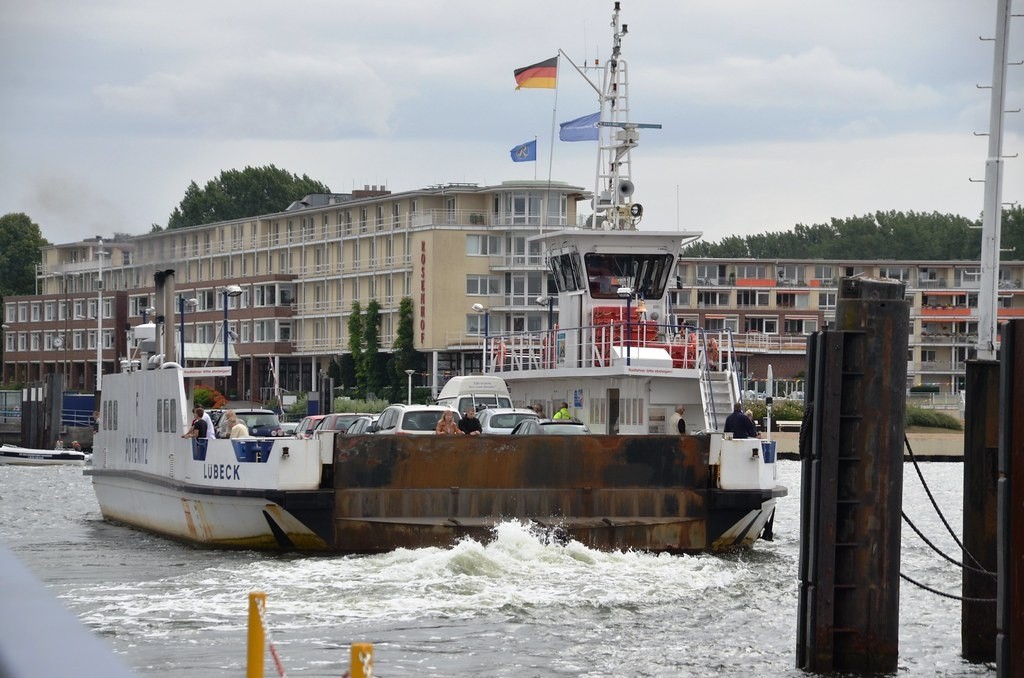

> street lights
[616,286,632,366]
[471,303,488,372]
[179,294,199,369]
[535,295,554,330]
[142,306,157,324]
[222,284,243,399]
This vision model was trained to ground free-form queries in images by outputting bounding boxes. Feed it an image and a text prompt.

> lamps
[282,447,290,459]
[227,330,239,340]
[623,202,643,231]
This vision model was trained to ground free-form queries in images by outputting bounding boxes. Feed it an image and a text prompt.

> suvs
[365,403,462,435]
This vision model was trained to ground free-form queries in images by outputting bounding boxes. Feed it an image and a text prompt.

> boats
[0,443,85,460]
[80,0,790,560]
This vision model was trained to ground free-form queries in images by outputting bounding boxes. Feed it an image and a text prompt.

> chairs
[336,423,346,430]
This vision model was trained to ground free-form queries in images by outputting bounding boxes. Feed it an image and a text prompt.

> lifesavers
[689,333,696,358]
[497,342,506,366]
[707,337,718,368]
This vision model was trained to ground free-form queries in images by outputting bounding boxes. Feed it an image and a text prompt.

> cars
[340,415,380,434]
[204,408,226,424]
[214,408,284,438]
[287,413,326,438]
[306,413,371,438]
[474,407,541,436]
[510,415,593,436]
[280,422,300,437]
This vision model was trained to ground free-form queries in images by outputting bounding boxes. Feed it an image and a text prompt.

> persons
[458,407,482,435]
[724,403,756,439]
[92,411,100,433]
[595,271,611,292]
[746,410,762,439]
[526,404,546,418]
[182,405,216,458]
[220,412,249,438]
[436,410,465,435]
[554,403,571,419]
[669,405,686,435]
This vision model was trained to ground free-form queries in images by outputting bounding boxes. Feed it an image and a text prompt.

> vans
[434,376,514,418]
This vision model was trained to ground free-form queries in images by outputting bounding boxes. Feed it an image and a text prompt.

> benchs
[776,420,802,432]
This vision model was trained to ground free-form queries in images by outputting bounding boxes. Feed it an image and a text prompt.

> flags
[560,111,601,141]
[510,140,536,162]
[514,57,557,91]
